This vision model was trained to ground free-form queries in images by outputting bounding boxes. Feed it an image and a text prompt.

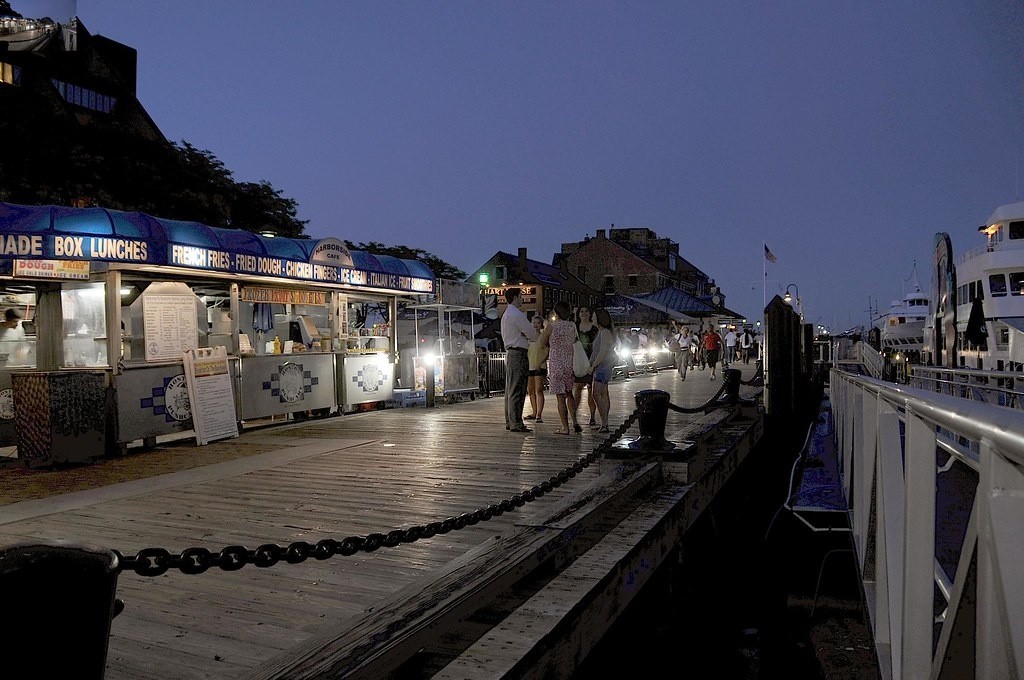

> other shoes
[506,425,527,430]
[710,376,712,380]
[510,428,530,433]
[589,418,595,425]
[597,425,610,433]
[713,375,716,379]
[681,377,684,381]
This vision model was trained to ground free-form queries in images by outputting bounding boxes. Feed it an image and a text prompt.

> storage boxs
[385,388,412,408]
[405,390,427,409]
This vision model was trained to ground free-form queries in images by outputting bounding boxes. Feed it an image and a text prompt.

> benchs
[611,360,630,380]
[632,355,659,375]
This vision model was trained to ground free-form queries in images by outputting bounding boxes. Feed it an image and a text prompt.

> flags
[765,244,776,263]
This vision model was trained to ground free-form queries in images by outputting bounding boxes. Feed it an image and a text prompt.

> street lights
[904,357,908,385]
[783,284,805,353]
[479,273,490,320]
[755,321,761,336]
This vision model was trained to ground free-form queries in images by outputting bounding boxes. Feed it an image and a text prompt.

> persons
[540,300,583,435]
[668,326,692,381]
[689,330,708,371]
[524,316,549,422]
[589,309,617,433]
[573,306,599,426]
[716,328,763,365]
[460,330,469,341]
[121,321,125,356]
[699,324,724,380]
[614,326,681,350]
[500,287,538,432]
[0,308,26,351]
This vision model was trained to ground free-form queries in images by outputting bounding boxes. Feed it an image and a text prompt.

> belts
[507,346,528,352]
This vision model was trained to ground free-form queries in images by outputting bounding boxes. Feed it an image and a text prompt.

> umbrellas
[474,318,502,339]
[445,311,486,335]
[964,297,989,357]
[408,318,461,336]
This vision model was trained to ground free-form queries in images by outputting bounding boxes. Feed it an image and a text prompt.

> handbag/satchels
[572,327,590,376]
[670,334,682,352]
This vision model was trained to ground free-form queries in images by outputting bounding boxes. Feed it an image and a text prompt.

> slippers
[553,429,568,435]
[574,424,582,434]
[535,417,543,423]
[523,416,536,420]
